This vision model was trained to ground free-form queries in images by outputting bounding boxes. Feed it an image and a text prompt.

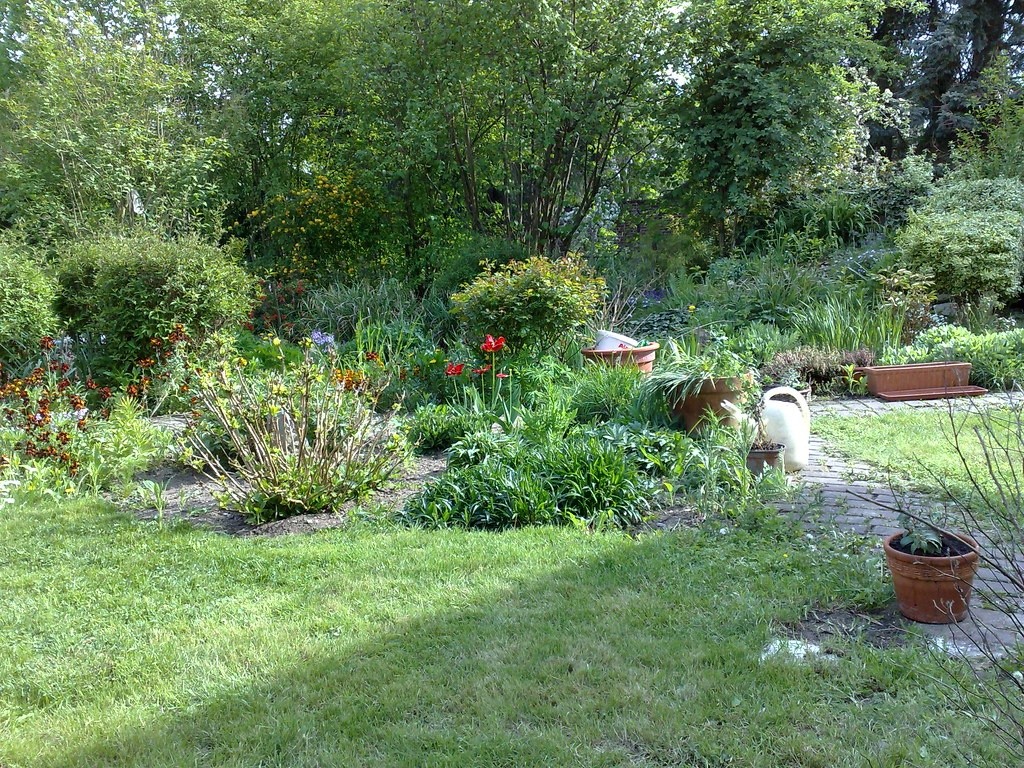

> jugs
[720,386,810,472]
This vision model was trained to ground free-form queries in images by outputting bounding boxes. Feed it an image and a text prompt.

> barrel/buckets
[592,330,638,350]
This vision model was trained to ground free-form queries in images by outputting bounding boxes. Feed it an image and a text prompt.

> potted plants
[662,332,752,439]
[883,484,980,624]
[726,357,786,480]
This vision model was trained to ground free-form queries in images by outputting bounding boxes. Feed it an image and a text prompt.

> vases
[593,330,638,350]
[865,361,973,398]
[581,342,660,379]
[843,367,868,388]
